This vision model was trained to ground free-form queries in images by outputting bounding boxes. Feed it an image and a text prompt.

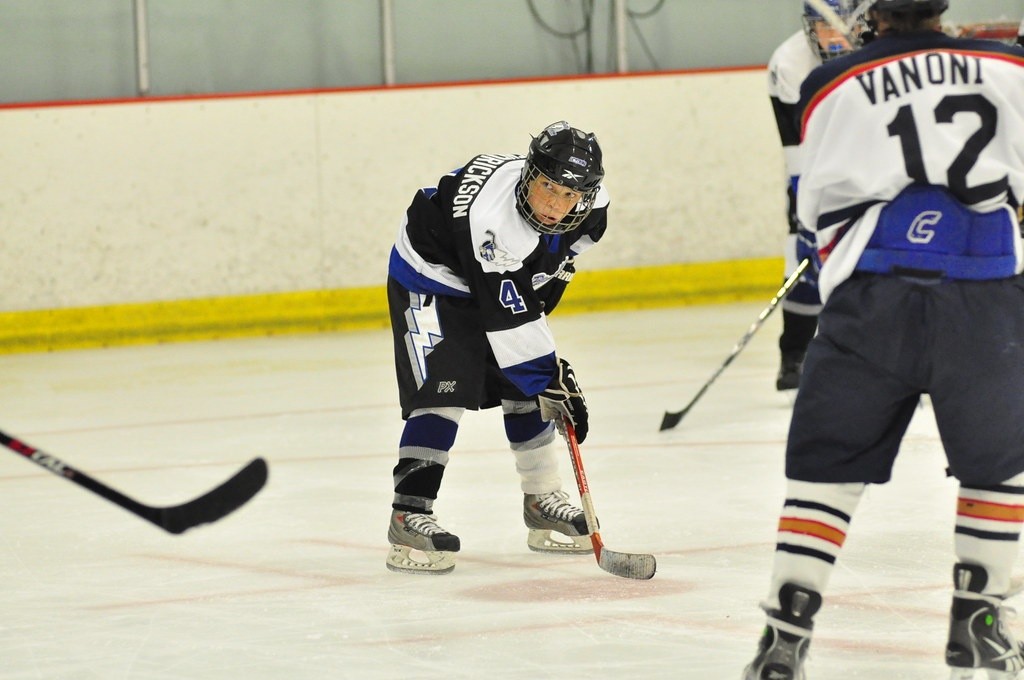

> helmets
[801,0,850,63]
[516,121,605,235]
[838,0,949,53]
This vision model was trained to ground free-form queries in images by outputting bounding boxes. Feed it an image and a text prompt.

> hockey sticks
[558,412,661,584]
[661,245,822,429]
[0,411,270,533]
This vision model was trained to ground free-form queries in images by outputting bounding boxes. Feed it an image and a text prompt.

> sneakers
[742,584,823,680]
[386,508,461,575]
[945,561,1024,680]
[776,348,803,404]
[524,489,600,553]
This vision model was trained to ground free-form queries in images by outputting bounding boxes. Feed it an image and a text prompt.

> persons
[743,0,1024,680]
[767,1,881,408]
[385,120,609,577]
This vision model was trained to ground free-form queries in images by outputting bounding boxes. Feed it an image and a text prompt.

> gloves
[535,265,577,317]
[539,358,589,444]
